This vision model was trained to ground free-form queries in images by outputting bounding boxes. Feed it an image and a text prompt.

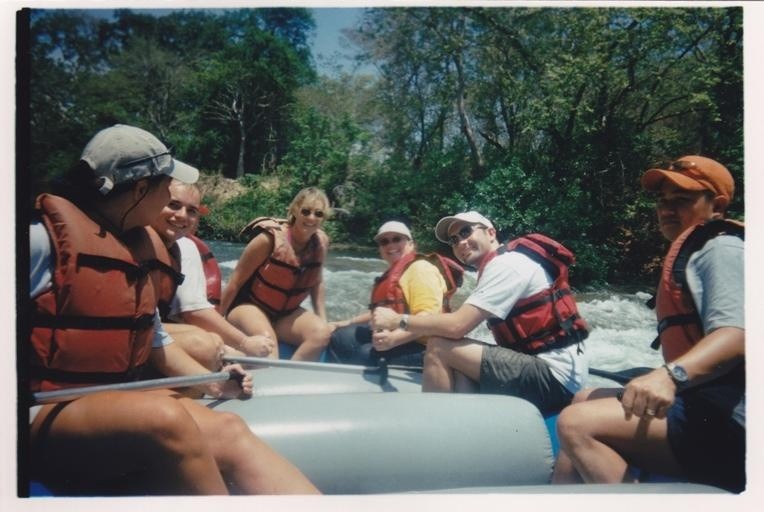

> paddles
[589,367,654,386]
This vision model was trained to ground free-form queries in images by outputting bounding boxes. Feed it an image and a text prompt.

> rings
[644,409,656,415]
[379,339,382,344]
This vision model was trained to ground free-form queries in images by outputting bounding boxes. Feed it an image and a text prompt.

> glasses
[652,160,697,173]
[447,225,487,246]
[302,208,324,218]
[378,236,402,244]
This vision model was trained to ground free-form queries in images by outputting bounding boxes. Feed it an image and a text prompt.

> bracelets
[235,336,247,351]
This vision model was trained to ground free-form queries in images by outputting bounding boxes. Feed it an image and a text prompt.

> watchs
[400,313,408,331]
[661,362,688,394]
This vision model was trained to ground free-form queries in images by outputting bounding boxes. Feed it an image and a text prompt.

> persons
[547,155,746,494]
[132,178,223,397]
[367,211,589,419]
[164,231,273,357]
[218,186,331,362]
[28,122,321,496]
[324,221,464,366]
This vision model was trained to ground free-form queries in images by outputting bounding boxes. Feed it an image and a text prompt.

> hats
[640,155,735,203]
[79,124,200,199]
[435,211,491,243]
[374,221,413,240]
